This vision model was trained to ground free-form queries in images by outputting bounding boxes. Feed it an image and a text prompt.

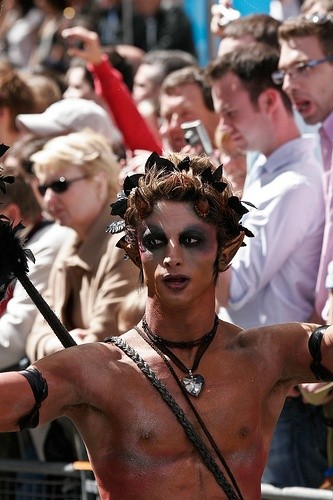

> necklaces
[142,311,220,398]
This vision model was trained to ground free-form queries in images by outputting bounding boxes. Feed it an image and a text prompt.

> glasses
[38,175,90,195]
[272,56,331,85]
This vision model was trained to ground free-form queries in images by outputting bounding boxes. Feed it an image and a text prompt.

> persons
[216,11,321,171]
[269,9,333,409]
[1,0,219,230]
[211,40,327,489]
[0,161,89,500]
[209,0,333,37]
[25,128,149,368]
[0,147,333,500]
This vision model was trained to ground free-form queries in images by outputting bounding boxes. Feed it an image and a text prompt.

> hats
[14,97,123,148]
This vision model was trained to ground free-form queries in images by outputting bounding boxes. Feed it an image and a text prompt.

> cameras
[181,120,213,156]
